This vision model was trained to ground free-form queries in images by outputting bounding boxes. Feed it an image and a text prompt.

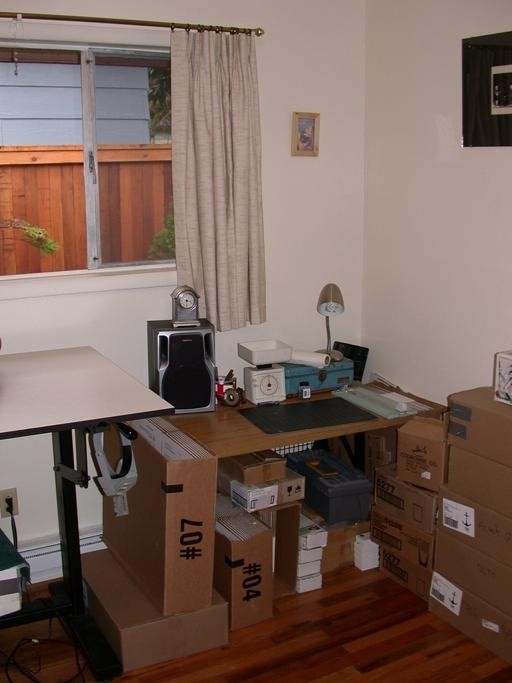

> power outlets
[0,488,19,518]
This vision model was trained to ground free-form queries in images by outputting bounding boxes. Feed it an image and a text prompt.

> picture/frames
[461,32,512,147]
[289,111,319,157]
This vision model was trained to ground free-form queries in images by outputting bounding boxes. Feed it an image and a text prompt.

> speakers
[146,318,217,414]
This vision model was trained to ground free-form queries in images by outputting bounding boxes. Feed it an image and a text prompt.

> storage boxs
[428,571,512,666]
[258,352,354,396]
[286,448,372,523]
[100,416,212,615]
[297,550,322,563]
[218,448,287,483]
[253,502,299,599]
[438,485,512,572]
[435,531,512,619]
[296,574,324,593]
[394,415,449,492]
[299,513,327,549]
[448,446,512,518]
[214,490,273,630]
[80,548,229,674]
[299,501,368,574]
[369,538,432,602]
[297,561,321,577]
[369,506,432,570]
[373,464,438,535]
[449,387,512,468]
[216,465,306,512]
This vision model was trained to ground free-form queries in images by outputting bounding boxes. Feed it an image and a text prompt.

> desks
[1,345,175,683]
[164,378,447,458]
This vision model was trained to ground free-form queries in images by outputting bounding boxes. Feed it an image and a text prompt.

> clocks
[172,286,200,327]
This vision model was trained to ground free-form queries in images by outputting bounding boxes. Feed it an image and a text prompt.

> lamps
[314,283,345,362]
